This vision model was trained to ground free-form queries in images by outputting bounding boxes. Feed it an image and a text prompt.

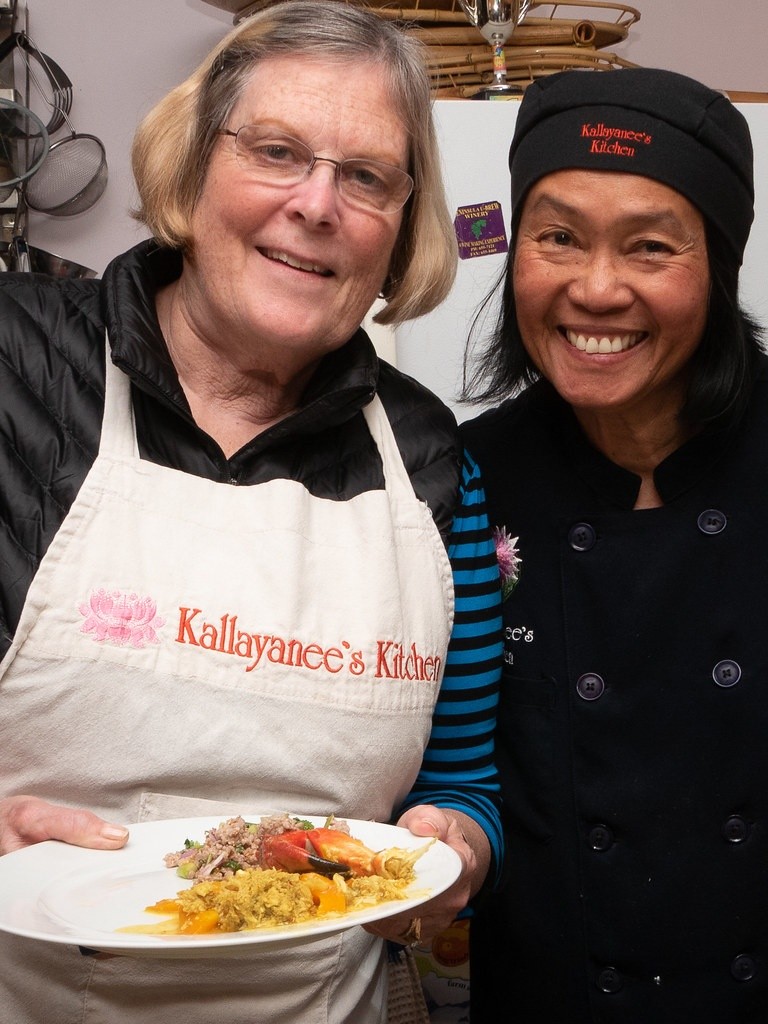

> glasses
[217,125,421,215]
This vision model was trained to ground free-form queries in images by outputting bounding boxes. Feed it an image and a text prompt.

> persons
[457,66,768,1024]
[0,0,505,1024]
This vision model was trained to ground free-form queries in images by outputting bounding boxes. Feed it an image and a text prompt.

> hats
[509,68,755,266]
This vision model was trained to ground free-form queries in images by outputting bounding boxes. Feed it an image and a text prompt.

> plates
[0,812,465,961]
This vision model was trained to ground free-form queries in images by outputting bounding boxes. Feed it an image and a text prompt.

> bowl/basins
[0,240,100,279]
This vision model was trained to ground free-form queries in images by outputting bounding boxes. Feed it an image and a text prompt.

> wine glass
[456,0,534,92]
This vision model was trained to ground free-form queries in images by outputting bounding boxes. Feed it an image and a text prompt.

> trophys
[458,0,534,101]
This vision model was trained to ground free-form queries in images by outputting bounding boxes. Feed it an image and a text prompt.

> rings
[402,918,423,952]
